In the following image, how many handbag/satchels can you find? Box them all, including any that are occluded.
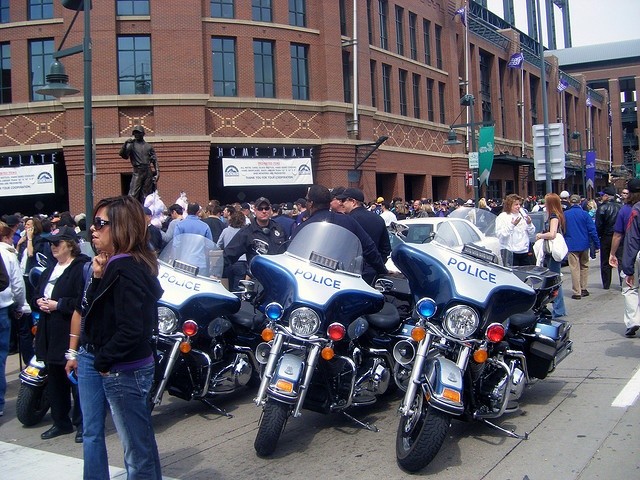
[543,226,563,255]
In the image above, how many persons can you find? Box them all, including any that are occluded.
[86,196,161,480]
[0,222,27,416]
[609,179,640,337]
[18,220,40,365]
[66,225,110,480]
[623,200,640,288]
[0,211,86,229]
[523,195,538,212]
[36,229,91,443]
[534,194,566,317]
[595,187,621,290]
[562,195,601,299]
[163,186,391,308]
[496,194,535,267]
[559,191,571,212]
[618,187,629,205]
[120,126,159,205]
[364,198,501,222]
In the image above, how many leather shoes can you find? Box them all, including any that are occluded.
[75,426,82,443]
[41,425,73,439]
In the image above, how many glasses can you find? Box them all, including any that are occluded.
[258,207,270,211]
[93,217,111,230]
[47,241,61,247]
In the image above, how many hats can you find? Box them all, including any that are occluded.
[377,197,384,203]
[133,125,144,134]
[336,188,365,202]
[7,215,20,226]
[42,226,77,242]
[598,187,615,196]
[570,194,582,203]
[255,197,270,207]
[53,211,60,217]
[560,190,570,198]
[272,204,280,213]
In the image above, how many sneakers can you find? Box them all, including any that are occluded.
[572,295,581,299]
[580,289,590,297]
[626,325,639,338]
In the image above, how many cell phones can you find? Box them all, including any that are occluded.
[67,370,78,385]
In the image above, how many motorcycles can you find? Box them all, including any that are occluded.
[12,251,53,428]
[153,233,268,420]
[391,234,572,472]
[250,220,412,455]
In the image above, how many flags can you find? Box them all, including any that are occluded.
[449,6,466,27]
[506,52,525,71]
[607,103,615,126]
[555,77,569,94]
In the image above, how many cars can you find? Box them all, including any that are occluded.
[385,218,504,274]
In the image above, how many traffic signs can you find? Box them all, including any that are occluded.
[532,123,567,181]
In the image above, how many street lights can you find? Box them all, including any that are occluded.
[35,0,94,240]
[565,132,586,198]
[442,94,478,208]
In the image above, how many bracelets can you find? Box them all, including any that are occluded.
[27,239,33,242]
[67,332,81,340]
[64,348,79,361]
